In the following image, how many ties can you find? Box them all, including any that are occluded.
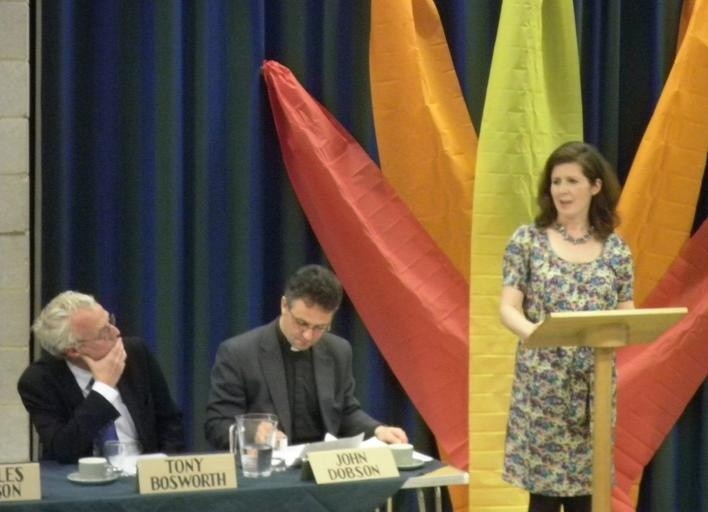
[87,378,119,456]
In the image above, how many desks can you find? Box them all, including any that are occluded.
[0,439,471,512]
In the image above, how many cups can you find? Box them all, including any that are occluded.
[76,455,108,478]
[103,438,143,472]
[391,444,414,465]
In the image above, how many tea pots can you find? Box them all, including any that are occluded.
[228,412,280,477]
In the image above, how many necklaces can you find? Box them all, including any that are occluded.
[555,223,595,245]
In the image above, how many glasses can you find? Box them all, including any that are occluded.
[77,313,116,342]
[291,312,329,332]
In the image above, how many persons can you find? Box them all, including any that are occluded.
[500,141,634,512]
[205,264,409,450]
[17,289,184,463]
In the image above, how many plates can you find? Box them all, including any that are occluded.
[395,458,424,472]
[67,468,119,486]
